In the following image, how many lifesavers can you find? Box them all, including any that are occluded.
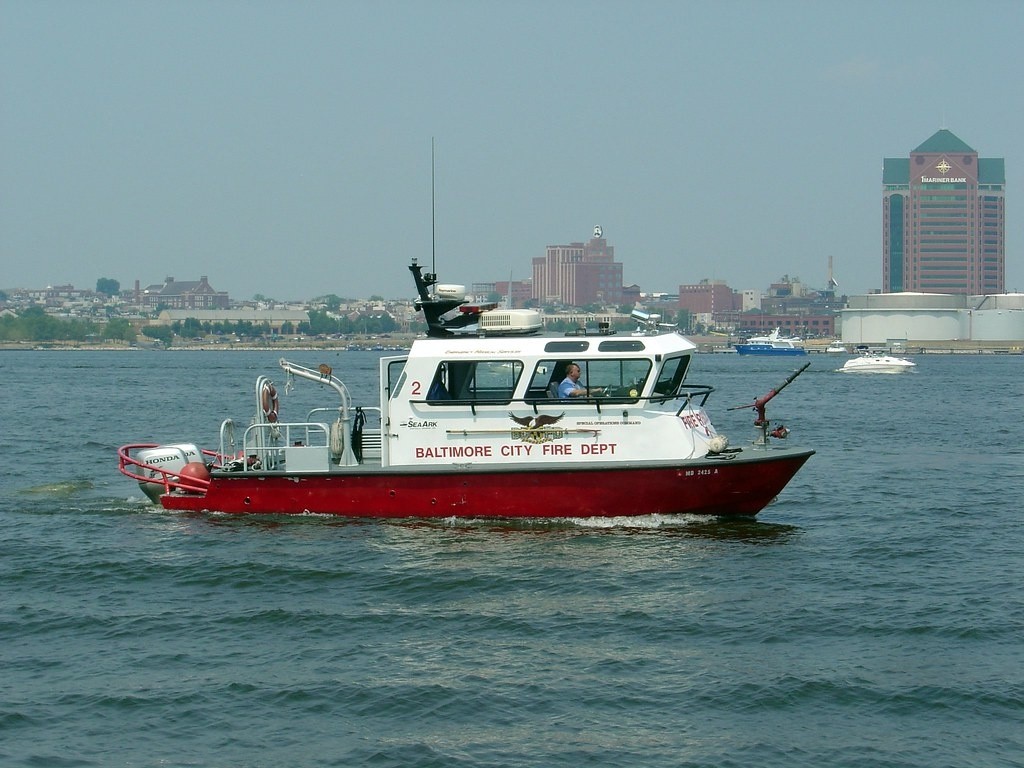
[262,384,279,421]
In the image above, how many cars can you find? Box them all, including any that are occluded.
[209,337,229,344]
[291,332,392,341]
[191,337,204,342]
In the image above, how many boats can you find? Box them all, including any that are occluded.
[842,342,917,375]
[117,136,816,520]
[827,339,847,353]
[731,324,808,357]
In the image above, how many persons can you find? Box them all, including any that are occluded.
[558,364,602,398]
